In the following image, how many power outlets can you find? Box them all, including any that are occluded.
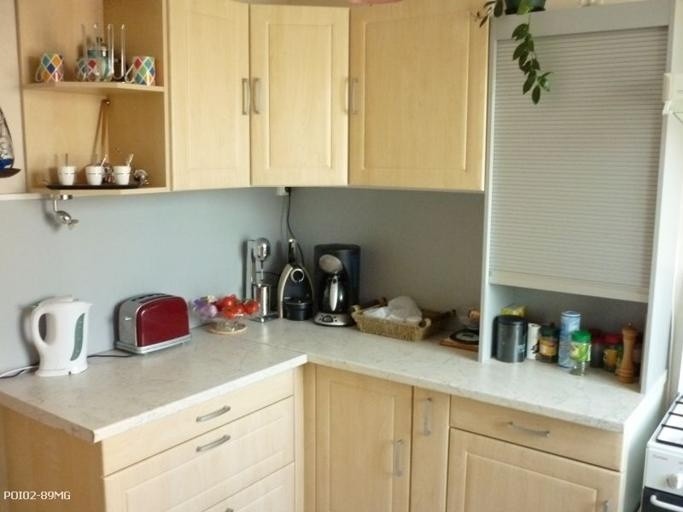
[277,186,293,197]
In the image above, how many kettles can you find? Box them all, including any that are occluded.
[24,294,92,376]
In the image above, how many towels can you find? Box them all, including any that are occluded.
[362,295,423,324]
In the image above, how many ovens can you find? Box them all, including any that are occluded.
[639,447,682,512]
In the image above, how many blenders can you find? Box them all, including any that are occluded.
[245,238,279,322]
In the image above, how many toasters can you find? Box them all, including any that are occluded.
[114,292,192,354]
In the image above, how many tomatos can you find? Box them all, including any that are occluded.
[216,296,259,315]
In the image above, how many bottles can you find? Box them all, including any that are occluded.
[558,311,581,367]
[616,322,637,378]
[632,332,644,376]
[588,329,603,367]
[539,327,558,362]
[570,332,589,376]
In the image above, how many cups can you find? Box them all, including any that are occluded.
[74,56,104,80]
[84,166,103,186]
[36,52,65,83]
[124,55,157,86]
[58,166,73,184]
[113,164,131,184]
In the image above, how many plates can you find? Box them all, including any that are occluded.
[450,329,479,344]
[81,185,111,188]
[46,184,81,189]
[112,184,138,190]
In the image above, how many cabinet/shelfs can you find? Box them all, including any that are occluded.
[17,0,171,197]
[104,0,347,193]
[348,0,489,194]
[0,355,308,512]
[306,353,667,512]
[477,1,683,393]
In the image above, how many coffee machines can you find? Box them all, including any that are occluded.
[313,245,360,327]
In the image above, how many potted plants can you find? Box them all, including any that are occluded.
[475,1,552,106]
